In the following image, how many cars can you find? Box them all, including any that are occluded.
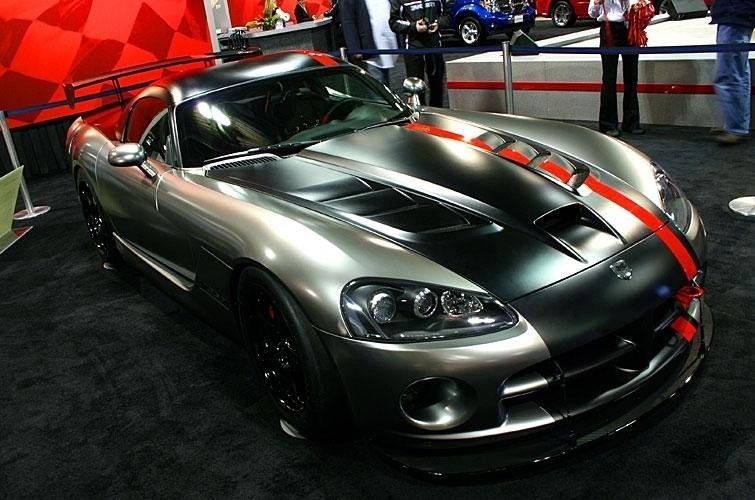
[438,2,535,45]
[532,1,602,28]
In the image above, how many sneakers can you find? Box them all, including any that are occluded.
[709,129,746,143]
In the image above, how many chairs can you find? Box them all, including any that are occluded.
[265,79,326,156]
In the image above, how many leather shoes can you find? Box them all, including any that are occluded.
[631,129,643,133]
[607,130,619,135]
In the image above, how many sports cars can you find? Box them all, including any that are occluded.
[63,41,716,481]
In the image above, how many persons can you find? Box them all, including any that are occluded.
[706,0,755,144]
[338,0,400,87]
[587,0,645,136]
[389,0,454,109]
[318,0,347,50]
[262,0,317,27]
